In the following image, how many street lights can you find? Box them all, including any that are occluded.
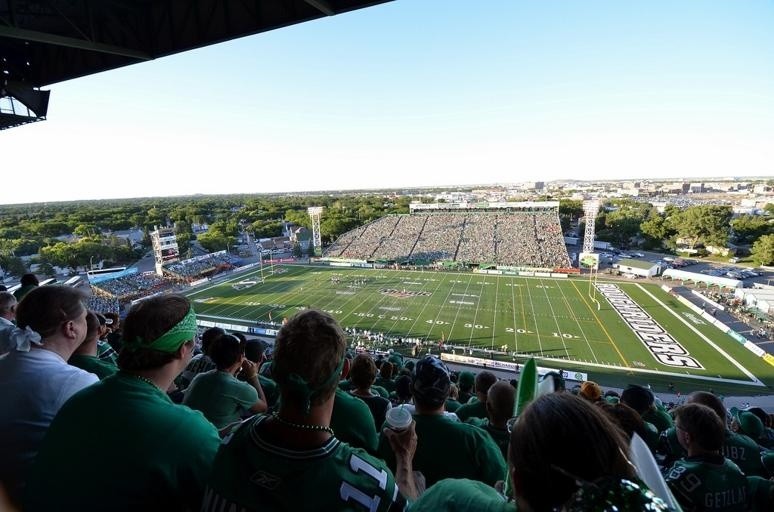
[89,256,95,280]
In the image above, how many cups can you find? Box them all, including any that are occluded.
[382,407,412,431]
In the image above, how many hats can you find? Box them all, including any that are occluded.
[729,406,765,440]
[457,370,475,387]
[412,354,451,404]
[580,380,602,400]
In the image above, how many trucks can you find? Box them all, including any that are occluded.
[564,236,581,247]
[593,240,615,252]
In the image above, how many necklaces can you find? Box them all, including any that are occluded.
[272,410,335,436]
[131,374,160,389]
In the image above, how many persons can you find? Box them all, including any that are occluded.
[3,287,102,508]
[67,311,124,381]
[698,283,772,338]
[209,311,417,512]
[18,295,221,509]
[295,309,773,512]
[267,311,273,321]
[3,275,39,355]
[328,276,340,284]
[79,277,206,316]
[164,327,280,440]
[349,278,369,292]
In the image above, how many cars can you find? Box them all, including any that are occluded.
[622,272,638,279]
[597,246,647,261]
[63,272,68,277]
[145,253,152,258]
[698,263,765,281]
[728,257,742,263]
[653,256,702,270]
[259,246,294,257]
